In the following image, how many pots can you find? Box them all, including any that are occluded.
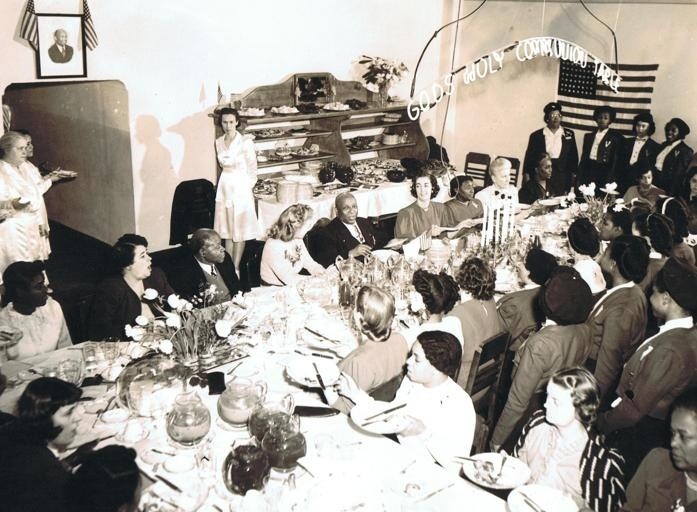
[382,132,399,146]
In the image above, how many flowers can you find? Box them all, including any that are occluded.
[559,178,620,226]
[119,283,255,395]
[358,54,411,105]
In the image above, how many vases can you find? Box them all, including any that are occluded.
[377,79,388,108]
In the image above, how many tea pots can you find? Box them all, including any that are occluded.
[162,368,306,498]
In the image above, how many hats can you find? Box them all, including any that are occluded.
[672,117,690,138]
[633,113,653,123]
[538,265,595,326]
[662,255,696,311]
[544,102,562,112]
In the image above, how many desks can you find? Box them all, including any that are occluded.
[254,156,467,244]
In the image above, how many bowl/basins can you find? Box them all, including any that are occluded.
[423,168,443,175]
[386,170,406,183]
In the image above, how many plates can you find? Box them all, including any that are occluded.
[254,193,273,200]
[276,179,313,205]
[0,325,22,346]
[284,352,577,511]
[238,113,266,119]
[271,112,301,117]
[292,151,317,158]
[321,108,348,113]
[255,134,283,138]
[287,131,306,136]
[84,398,164,464]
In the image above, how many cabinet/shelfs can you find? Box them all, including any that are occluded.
[208,72,430,186]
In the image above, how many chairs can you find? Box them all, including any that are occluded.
[0,152,522,344]
[363,274,671,500]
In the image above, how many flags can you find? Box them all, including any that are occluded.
[557,61,660,137]
[20,0,39,52]
[83,0,99,50]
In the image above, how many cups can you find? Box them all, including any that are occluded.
[334,232,524,328]
[56,334,120,386]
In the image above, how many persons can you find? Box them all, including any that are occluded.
[49,29,73,63]
[3,103,697,512]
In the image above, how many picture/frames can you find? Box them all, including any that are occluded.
[34,12,88,76]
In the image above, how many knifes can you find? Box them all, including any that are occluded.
[303,326,336,343]
